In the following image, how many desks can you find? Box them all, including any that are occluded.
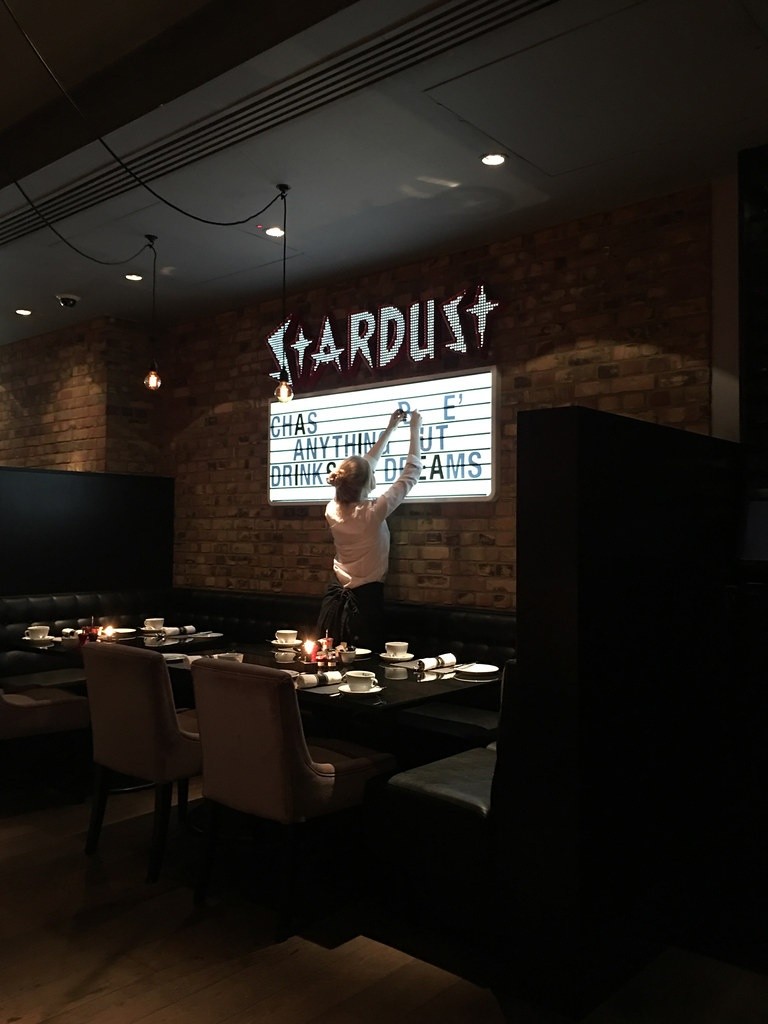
[109,633,503,845]
[6,610,215,792]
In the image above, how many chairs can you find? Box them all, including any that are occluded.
[80,637,200,880]
[187,656,401,944]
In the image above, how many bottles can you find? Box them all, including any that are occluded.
[328,652,336,670]
[316,652,325,673]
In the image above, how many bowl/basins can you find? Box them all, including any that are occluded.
[355,648,372,659]
[113,629,136,637]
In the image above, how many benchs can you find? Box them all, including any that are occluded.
[0,586,511,948]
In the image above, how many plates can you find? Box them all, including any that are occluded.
[337,685,381,694]
[380,653,414,662]
[22,636,54,640]
[112,637,136,640]
[276,661,295,663]
[141,628,163,632]
[271,640,302,648]
[454,663,499,674]
[188,633,223,637]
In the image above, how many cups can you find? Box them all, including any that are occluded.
[144,618,164,629]
[144,637,158,645]
[275,630,298,644]
[346,671,378,691]
[218,653,243,664]
[275,652,295,661]
[25,626,50,639]
[385,642,408,657]
[340,652,356,663]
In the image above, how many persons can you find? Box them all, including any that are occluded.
[309,409,422,755]
